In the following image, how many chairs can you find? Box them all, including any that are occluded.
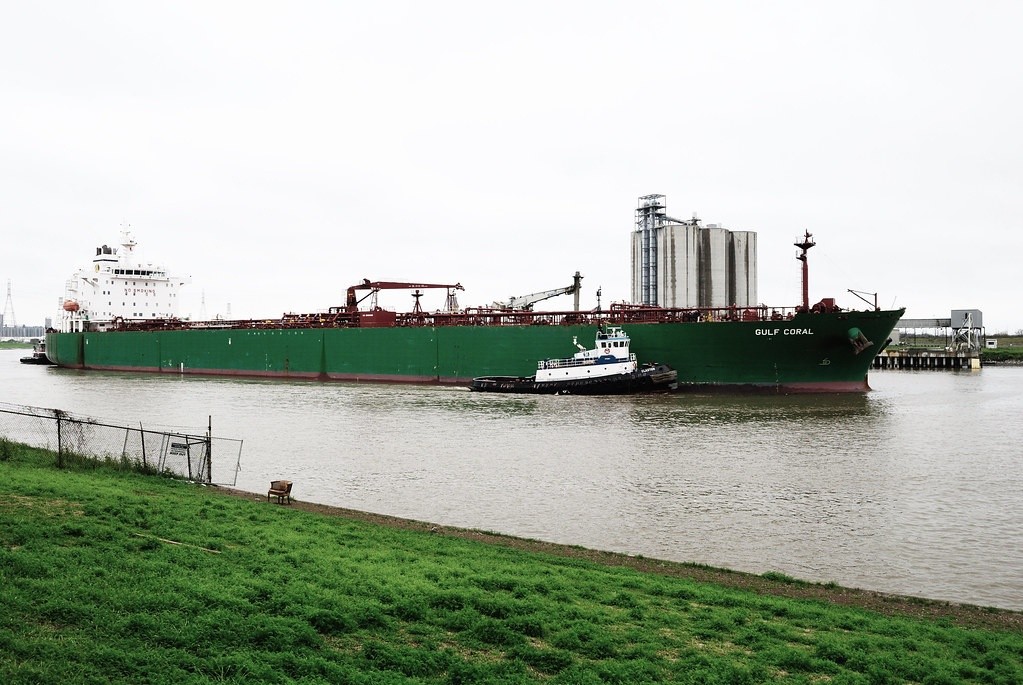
[268,479,292,505]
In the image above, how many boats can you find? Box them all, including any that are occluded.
[467,323,680,395]
[42,221,906,392]
[18,338,54,365]
